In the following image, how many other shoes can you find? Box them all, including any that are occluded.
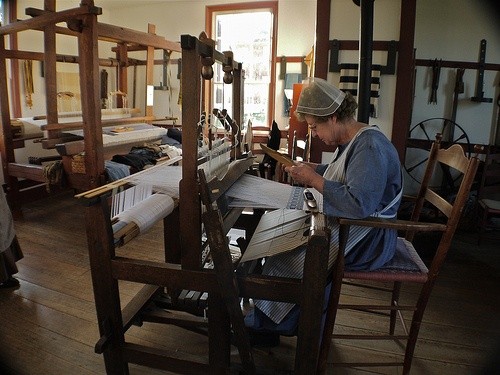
[198,300,208,317]
[229,326,279,347]
[4,278,19,287]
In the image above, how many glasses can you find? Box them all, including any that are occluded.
[309,115,325,131]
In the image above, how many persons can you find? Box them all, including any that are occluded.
[0,183,25,287]
[230,76,405,348]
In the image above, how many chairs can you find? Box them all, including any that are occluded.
[248,120,281,180]
[290,130,309,187]
[474,164,500,247]
[317,133,484,375]
